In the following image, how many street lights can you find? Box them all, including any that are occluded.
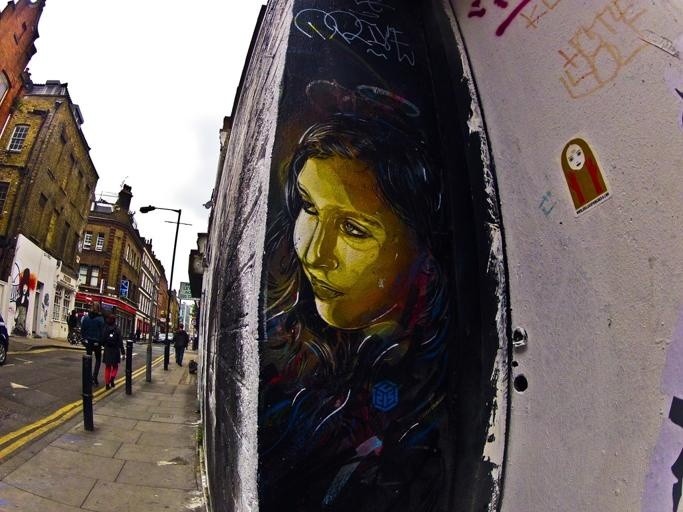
[139,206,181,345]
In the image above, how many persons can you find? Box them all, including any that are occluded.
[80,302,103,387]
[265,110,461,511]
[67,308,78,345]
[14,277,30,314]
[102,314,126,390]
[172,323,188,364]
[74,310,89,327]
[135,327,140,342]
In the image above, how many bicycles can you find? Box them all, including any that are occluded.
[67,327,80,345]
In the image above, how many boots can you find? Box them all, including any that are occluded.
[106,377,115,389]
[92,375,100,384]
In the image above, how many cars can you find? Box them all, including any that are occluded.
[155,333,166,343]
[167,332,174,343]
[0,314,8,363]
